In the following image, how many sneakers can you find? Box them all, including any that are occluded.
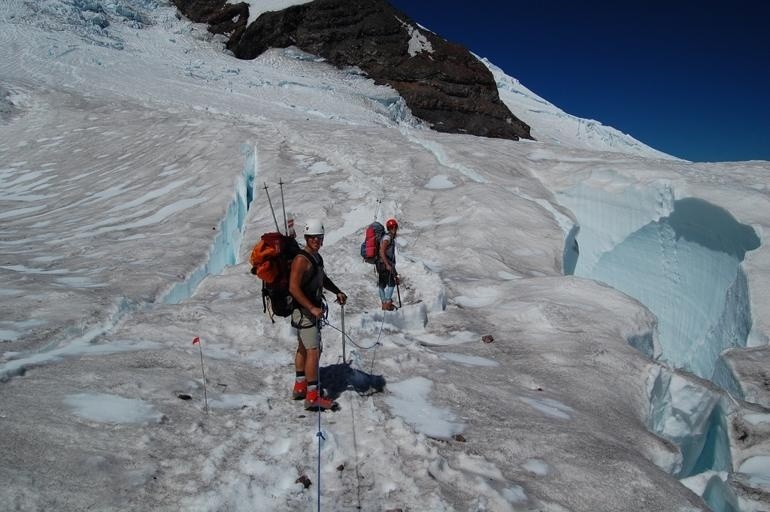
[382,303,398,310]
[294,380,339,408]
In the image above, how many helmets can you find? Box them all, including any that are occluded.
[304,220,324,235]
[386,219,398,230]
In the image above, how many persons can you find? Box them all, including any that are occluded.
[285,219,346,409]
[376,219,400,312]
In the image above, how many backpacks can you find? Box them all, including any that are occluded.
[250,233,324,317]
[360,222,393,264]
